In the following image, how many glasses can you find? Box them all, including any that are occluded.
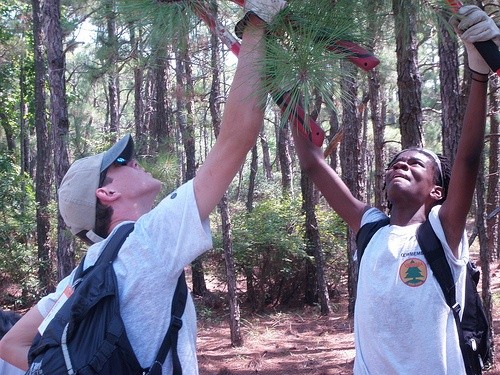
[57,133,135,236]
[99,154,128,188]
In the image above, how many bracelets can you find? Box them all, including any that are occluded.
[471,77,489,83]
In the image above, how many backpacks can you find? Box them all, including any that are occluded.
[24,222,188,375]
[354,217,494,374]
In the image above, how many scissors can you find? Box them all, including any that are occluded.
[187,0,380,147]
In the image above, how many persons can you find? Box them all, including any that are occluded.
[288,6,500,375]
[0,0,289,374]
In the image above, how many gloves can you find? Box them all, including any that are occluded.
[448,4,500,75]
[234,0,288,40]
